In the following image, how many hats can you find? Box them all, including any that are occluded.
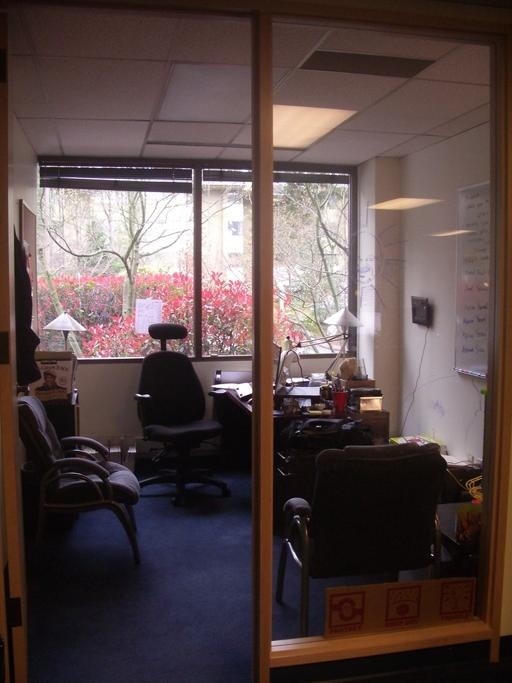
[43,370,56,376]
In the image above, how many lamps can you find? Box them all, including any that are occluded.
[276,304,364,408]
[43,310,88,350]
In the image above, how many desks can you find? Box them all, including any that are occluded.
[219,372,390,530]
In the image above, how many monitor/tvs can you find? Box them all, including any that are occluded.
[235,340,282,400]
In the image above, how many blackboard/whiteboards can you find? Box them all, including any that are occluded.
[452,180,491,379]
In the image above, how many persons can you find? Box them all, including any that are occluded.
[34,370,68,391]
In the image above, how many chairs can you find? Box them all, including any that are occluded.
[136,322,237,507]
[276,441,449,637]
[17,395,146,563]
